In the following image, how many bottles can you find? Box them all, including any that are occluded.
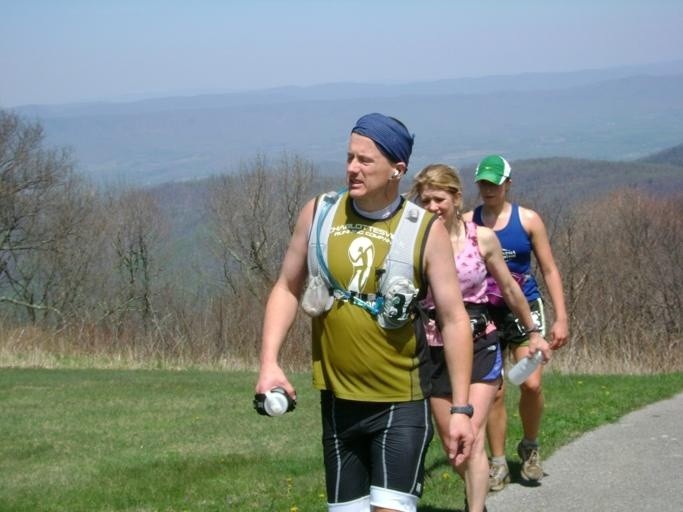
[507,350,547,387]
[261,388,295,417]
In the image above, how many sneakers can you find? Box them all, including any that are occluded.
[517,440,543,482]
[489,460,511,491]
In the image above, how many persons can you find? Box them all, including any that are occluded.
[254,113,476,512]
[460,154,571,493]
[408,162,553,512]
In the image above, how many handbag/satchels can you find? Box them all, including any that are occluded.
[430,301,491,338]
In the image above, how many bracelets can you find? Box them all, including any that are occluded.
[523,324,539,334]
[448,403,474,419]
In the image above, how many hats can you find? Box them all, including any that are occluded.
[474,155,511,185]
